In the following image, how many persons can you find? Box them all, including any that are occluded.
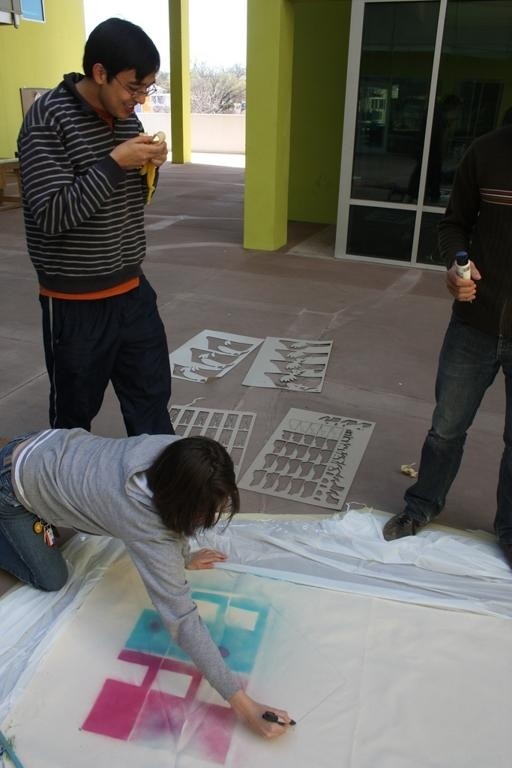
[382,120,512,572]
[0,426,292,741]
[401,117,451,263]
[16,18,177,437]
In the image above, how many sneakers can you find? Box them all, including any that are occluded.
[383,511,422,540]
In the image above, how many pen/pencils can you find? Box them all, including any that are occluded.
[454,252,471,301]
[263,711,297,724]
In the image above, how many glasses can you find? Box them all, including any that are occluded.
[114,77,157,97]
